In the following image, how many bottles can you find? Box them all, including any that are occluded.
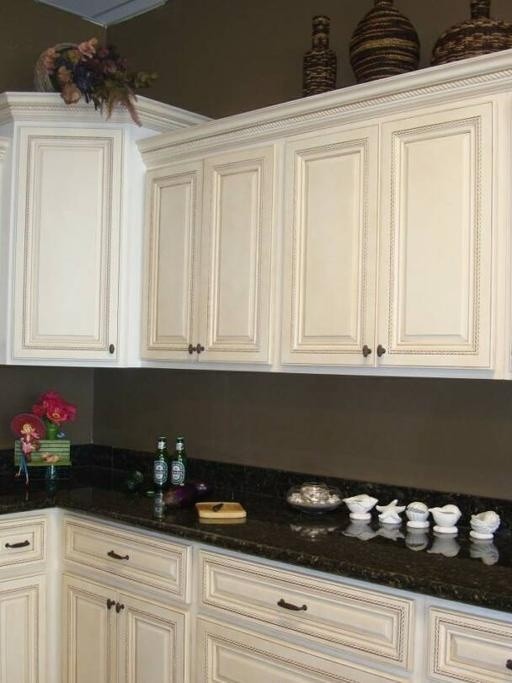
[169,431,188,490]
[153,491,168,521]
[153,432,168,490]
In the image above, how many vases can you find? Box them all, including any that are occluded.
[46,422,58,436]
[302,15,337,97]
[32,42,79,92]
[430,0,512,67]
[349,0,421,84]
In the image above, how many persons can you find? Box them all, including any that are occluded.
[11,412,45,485]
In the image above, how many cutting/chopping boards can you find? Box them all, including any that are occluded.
[194,502,247,518]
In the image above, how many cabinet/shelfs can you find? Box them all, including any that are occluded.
[284,51,503,381]
[60,519,193,683]
[195,545,420,683]
[0,91,128,370]
[0,515,53,683]
[140,103,284,373]
[422,602,512,683]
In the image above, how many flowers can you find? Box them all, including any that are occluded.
[31,388,79,440]
[43,37,161,128]
